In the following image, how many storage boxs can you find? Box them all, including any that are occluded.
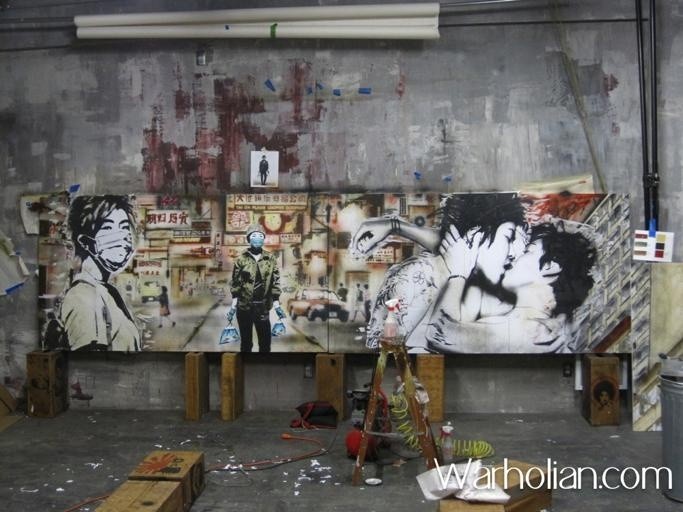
[94,450,205,512]
[439,460,554,512]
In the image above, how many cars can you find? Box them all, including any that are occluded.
[287,289,348,322]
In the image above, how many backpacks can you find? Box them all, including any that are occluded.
[41,268,112,351]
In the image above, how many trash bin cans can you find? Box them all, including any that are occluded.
[659,375,683,502]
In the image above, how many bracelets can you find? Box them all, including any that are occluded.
[387,214,400,233]
[445,275,468,280]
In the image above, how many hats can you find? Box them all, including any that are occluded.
[247,224,267,238]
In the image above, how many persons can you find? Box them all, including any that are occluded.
[346,213,597,356]
[257,153,269,185]
[361,283,371,323]
[361,193,574,357]
[348,281,365,322]
[593,381,614,407]
[156,285,177,329]
[223,222,286,352]
[44,194,147,355]
[337,282,348,302]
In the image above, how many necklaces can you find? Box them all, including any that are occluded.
[512,304,552,318]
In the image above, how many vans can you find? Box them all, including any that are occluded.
[140,275,163,302]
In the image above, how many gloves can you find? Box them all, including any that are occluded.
[227,308,236,320]
[275,307,286,318]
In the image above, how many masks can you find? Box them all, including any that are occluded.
[77,231,135,273]
[250,238,264,248]
